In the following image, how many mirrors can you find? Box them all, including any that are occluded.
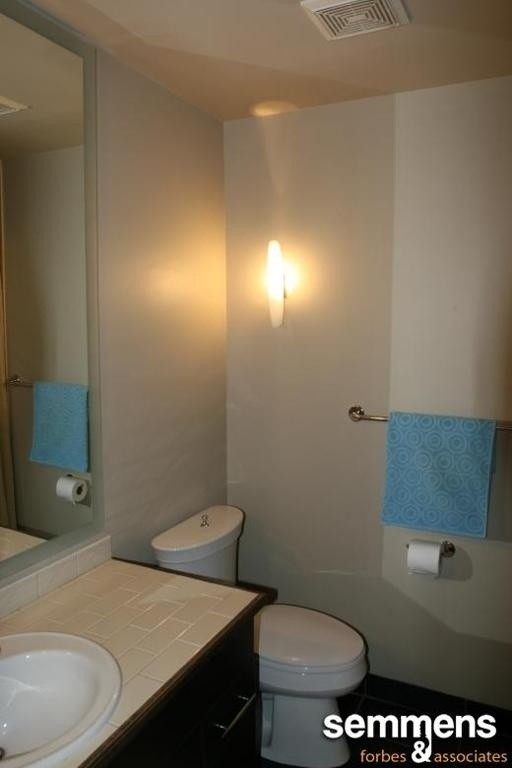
[1,0,107,592]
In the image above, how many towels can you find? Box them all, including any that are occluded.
[378,410,498,539]
[29,379,91,474]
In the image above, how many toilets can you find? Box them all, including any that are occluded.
[151,505,369,768]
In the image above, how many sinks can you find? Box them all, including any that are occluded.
[1,631,122,766]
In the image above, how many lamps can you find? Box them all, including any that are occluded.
[265,239,288,327]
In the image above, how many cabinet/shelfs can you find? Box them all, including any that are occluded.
[106,618,265,767]
